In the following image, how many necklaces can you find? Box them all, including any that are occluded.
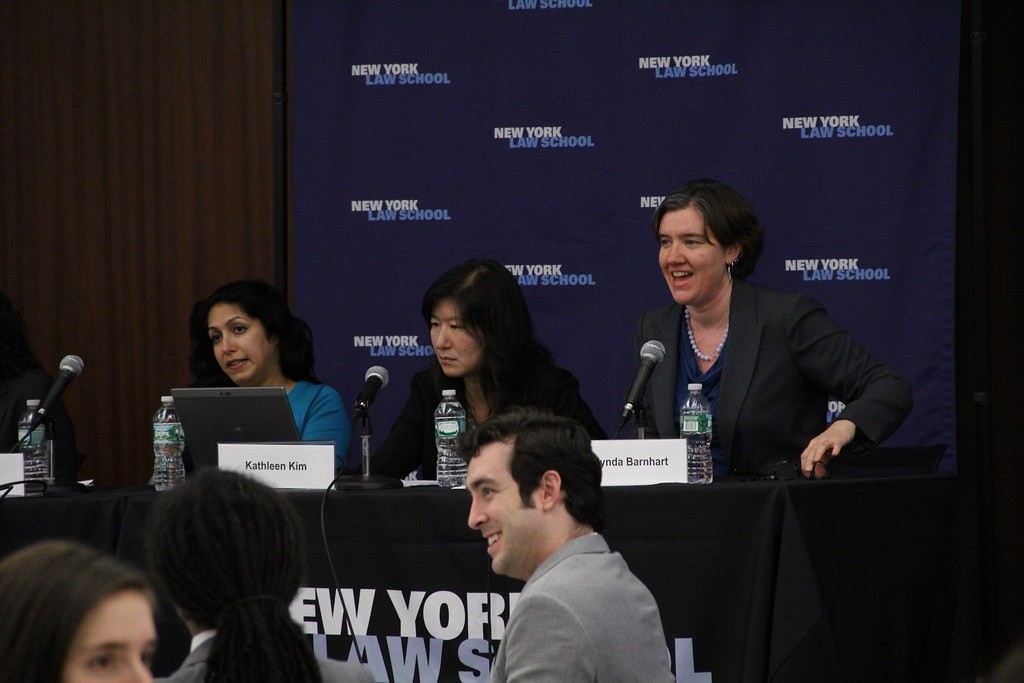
[684,308,730,361]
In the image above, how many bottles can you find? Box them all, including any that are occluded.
[682,382,716,485]
[431,389,469,485]
[17,397,49,484]
[151,394,184,493]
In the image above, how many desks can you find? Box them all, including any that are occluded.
[0,474,972,682]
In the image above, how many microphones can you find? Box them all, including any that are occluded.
[352,365,389,425]
[30,355,86,431]
[616,340,666,428]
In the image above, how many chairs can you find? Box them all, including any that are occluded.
[862,443,947,475]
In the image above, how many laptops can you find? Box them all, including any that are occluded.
[170,386,299,473]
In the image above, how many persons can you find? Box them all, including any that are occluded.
[458,407,677,681]
[145,468,380,682]
[629,180,914,480]
[0,540,158,683]
[351,258,611,484]
[146,275,351,491]
[1,290,79,488]
